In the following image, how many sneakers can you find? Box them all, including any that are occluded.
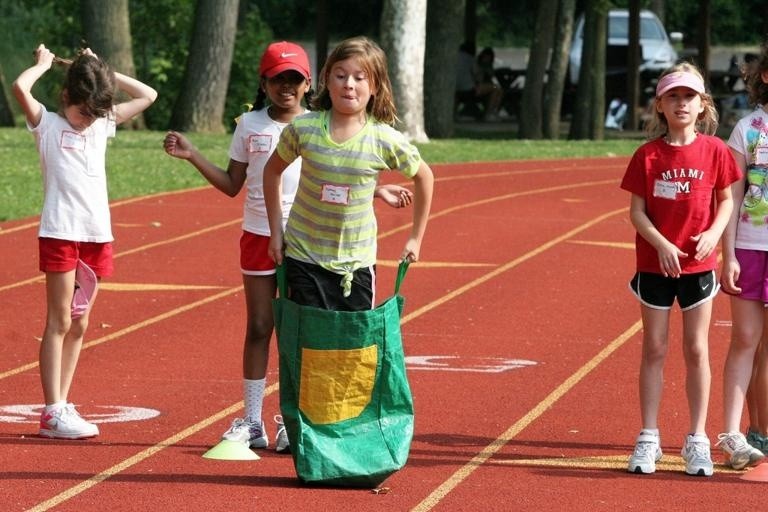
[274,414,291,454]
[628,427,768,477]
[222,416,268,448]
[39,403,99,439]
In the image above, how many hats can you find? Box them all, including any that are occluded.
[260,41,309,83]
[71,259,97,320]
[655,72,705,96]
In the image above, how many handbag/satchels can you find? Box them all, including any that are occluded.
[273,298,414,487]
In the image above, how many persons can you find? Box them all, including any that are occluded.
[474,46,503,123]
[719,49,768,470]
[11,43,159,439]
[722,53,757,110]
[262,36,435,487]
[454,39,479,123]
[163,39,415,453]
[619,62,745,476]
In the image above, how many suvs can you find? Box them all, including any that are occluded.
[569,7,678,90]
